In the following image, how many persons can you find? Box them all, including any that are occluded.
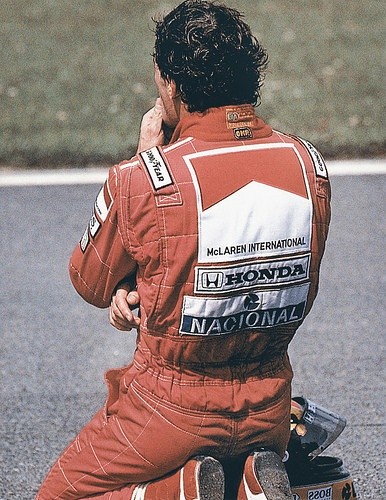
[37,0,333,500]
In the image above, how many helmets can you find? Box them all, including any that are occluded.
[289,396,356,500]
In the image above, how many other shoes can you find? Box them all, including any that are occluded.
[181,454,225,500]
[242,449,294,499]
